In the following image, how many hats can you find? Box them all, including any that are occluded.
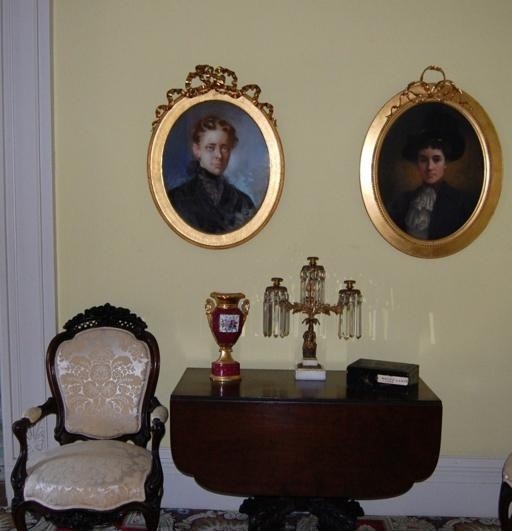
[401,127,463,164]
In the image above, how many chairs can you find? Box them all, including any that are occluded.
[9,304,168,531]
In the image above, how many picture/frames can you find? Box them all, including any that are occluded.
[147,65,285,251]
[358,66,502,262]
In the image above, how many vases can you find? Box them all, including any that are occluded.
[206,292,250,383]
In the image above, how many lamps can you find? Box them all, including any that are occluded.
[264,256,363,383]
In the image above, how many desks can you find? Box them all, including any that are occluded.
[168,368,442,531]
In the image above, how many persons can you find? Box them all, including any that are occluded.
[164,113,255,234]
[389,128,475,241]
